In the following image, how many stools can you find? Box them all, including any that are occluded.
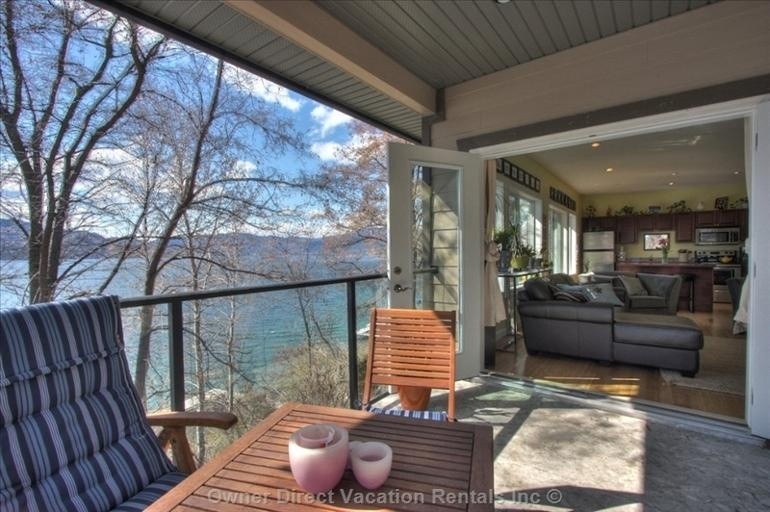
[670,272,697,314]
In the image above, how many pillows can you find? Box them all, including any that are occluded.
[581,282,625,307]
[618,274,649,296]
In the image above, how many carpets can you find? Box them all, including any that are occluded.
[659,335,745,396]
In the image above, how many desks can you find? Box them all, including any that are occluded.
[493,265,554,354]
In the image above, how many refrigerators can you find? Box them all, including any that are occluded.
[581,230,615,272]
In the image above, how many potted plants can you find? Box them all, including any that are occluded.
[492,223,553,273]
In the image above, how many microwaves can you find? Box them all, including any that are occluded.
[696,227,741,246]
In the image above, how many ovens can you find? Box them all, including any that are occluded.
[712,263,743,304]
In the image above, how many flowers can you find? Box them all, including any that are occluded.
[658,239,671,247]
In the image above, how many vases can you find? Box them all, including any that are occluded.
[662,247,669,263]
[397,386,431,411]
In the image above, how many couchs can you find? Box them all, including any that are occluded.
[517,269,704,379]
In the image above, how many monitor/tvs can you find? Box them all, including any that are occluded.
[643,233,670,251]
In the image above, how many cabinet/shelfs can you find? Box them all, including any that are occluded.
[739,209,748,240]
[675,213,695,242]
[637,214,674,231]
[587,217,616,230]
[695,209,738,227]
[617,215,637,242]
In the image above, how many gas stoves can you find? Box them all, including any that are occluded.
[698,255,739,265]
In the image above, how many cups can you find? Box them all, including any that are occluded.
[286,421,350,497]
[350,440,394,491]
